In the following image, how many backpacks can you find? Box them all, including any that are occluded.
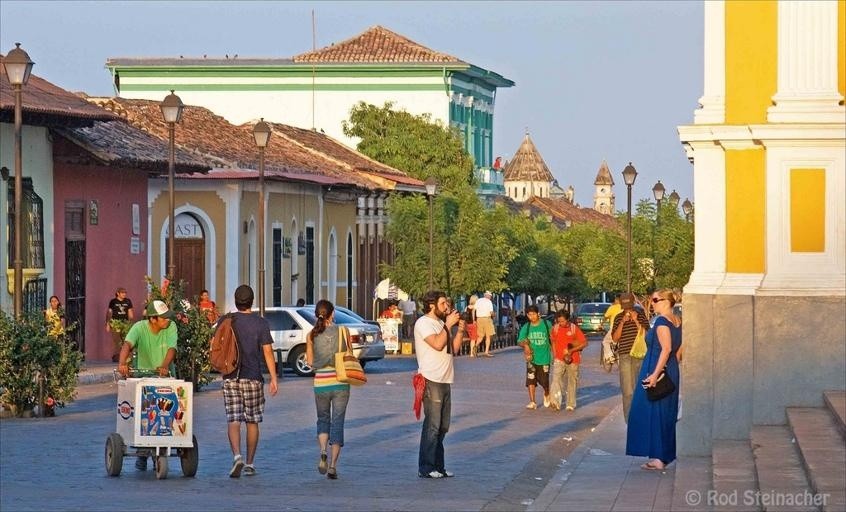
[209,313,240,375]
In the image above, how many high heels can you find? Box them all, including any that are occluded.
[317,450,327,475]
[326,467,339,478]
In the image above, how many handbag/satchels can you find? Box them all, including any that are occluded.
[334,326,367,386]
[630,324,648,359]
[646,318,675,401]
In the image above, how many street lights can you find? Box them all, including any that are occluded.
[682,198,694,227]
[653,180,664,291]
[159,88,185,310]
[424,175,438,293]
[4,40,34,322]
[668,189,680,222]
[253,116,272,319]
[622,162,638,295]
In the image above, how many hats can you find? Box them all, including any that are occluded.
[619,292,636,309]
[484,289,493,297]
[146,300,178,322]
[235,284,253,303]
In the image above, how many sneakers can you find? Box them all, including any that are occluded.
[134,456,146,472]
[418,469,444,479]
[566,405,576,414]
[527,402,538,411]
[229,456,244,478]
[153,460,159,470]
[439,467,453,478]
[542,396,550,407]
[550,402,561,415]
[243,466,258,476]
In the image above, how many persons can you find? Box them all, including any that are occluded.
[465,295,478,358]
[550,310,588,410]
[415,291,464,478]
[517,305,552,409]
[626,287,683,470]
[398,299,416,339]
[296,299,303,307]
[200,290,220,328]
[383,304,400,319]
[106,288,134,362]
[211,286,277,478]
[612,294,650,424]
[45,296,66,336]
[119,301,178,469]
[307,300,353,478]
[473,291,496,357]
[599,294,624,366]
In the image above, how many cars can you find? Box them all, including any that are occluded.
[574,303,611,336]
[212,307,385,377]
[305,305,381,328]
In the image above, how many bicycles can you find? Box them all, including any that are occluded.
[600,327,619,373]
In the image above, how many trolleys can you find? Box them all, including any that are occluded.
[105,368,198,480]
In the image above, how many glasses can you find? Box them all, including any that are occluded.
[652,296,669,303]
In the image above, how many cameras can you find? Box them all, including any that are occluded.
[450,307,476,326]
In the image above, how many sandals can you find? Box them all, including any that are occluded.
[473,346,479,355]
[485,353,493,356]
[641,459,669,472]
